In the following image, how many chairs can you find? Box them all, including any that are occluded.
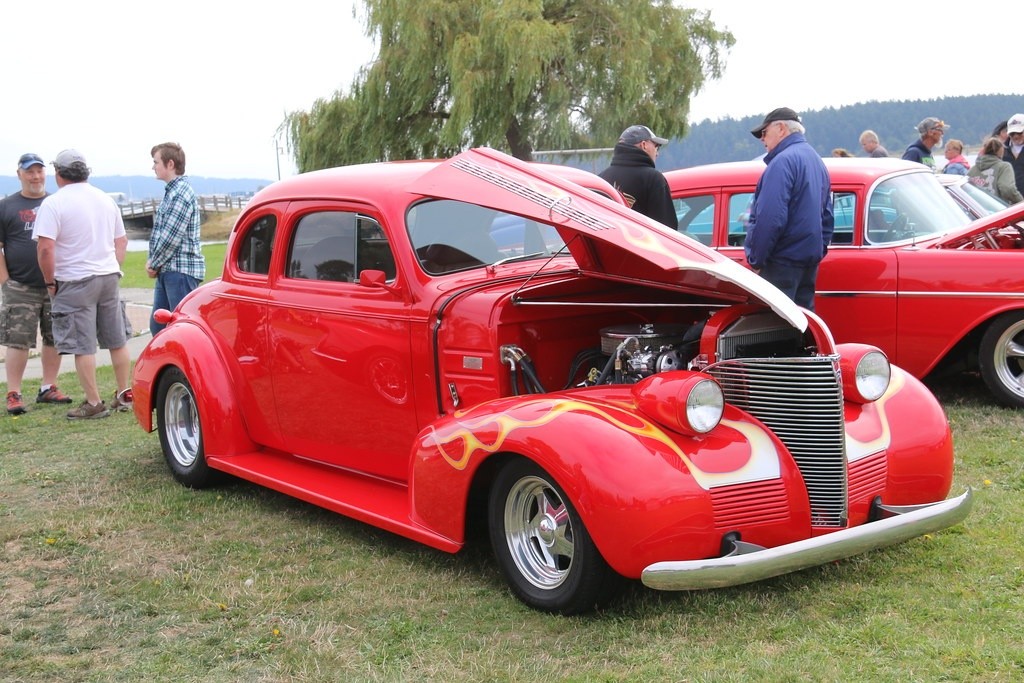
[292,212,509,283]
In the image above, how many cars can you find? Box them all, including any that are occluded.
[660,158,1023,409]
[132,146,973,616]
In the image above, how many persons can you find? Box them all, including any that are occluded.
[594,124,677,234]
[967,138,1023,205]
[743,107,835,310]
[0,152,73,413]
[832,148,854,157]
[977,121,1008,159]
[31,150,132,419]
[895,117,950,233]
[1001,114,1024,198]
[941,139,971,175]
[145,142,207,339]
[859,130,888,157]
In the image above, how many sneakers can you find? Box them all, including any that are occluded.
[7,390,23,412]
[67,400,109,420]
[36,388,72,403]
[110,391,132,408]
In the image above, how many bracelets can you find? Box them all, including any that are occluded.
[44,282,55,286]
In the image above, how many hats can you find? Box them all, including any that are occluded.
[50,148,86,168]
[918,117,951,132]
[618,125,669,145]
[17,153,45,169]
[751,107,800,138]
[1007,114,1024,135]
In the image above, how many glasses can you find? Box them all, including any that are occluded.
[932,121,944,129]
[1010,133,1023,136]
[935,128,943,135]
